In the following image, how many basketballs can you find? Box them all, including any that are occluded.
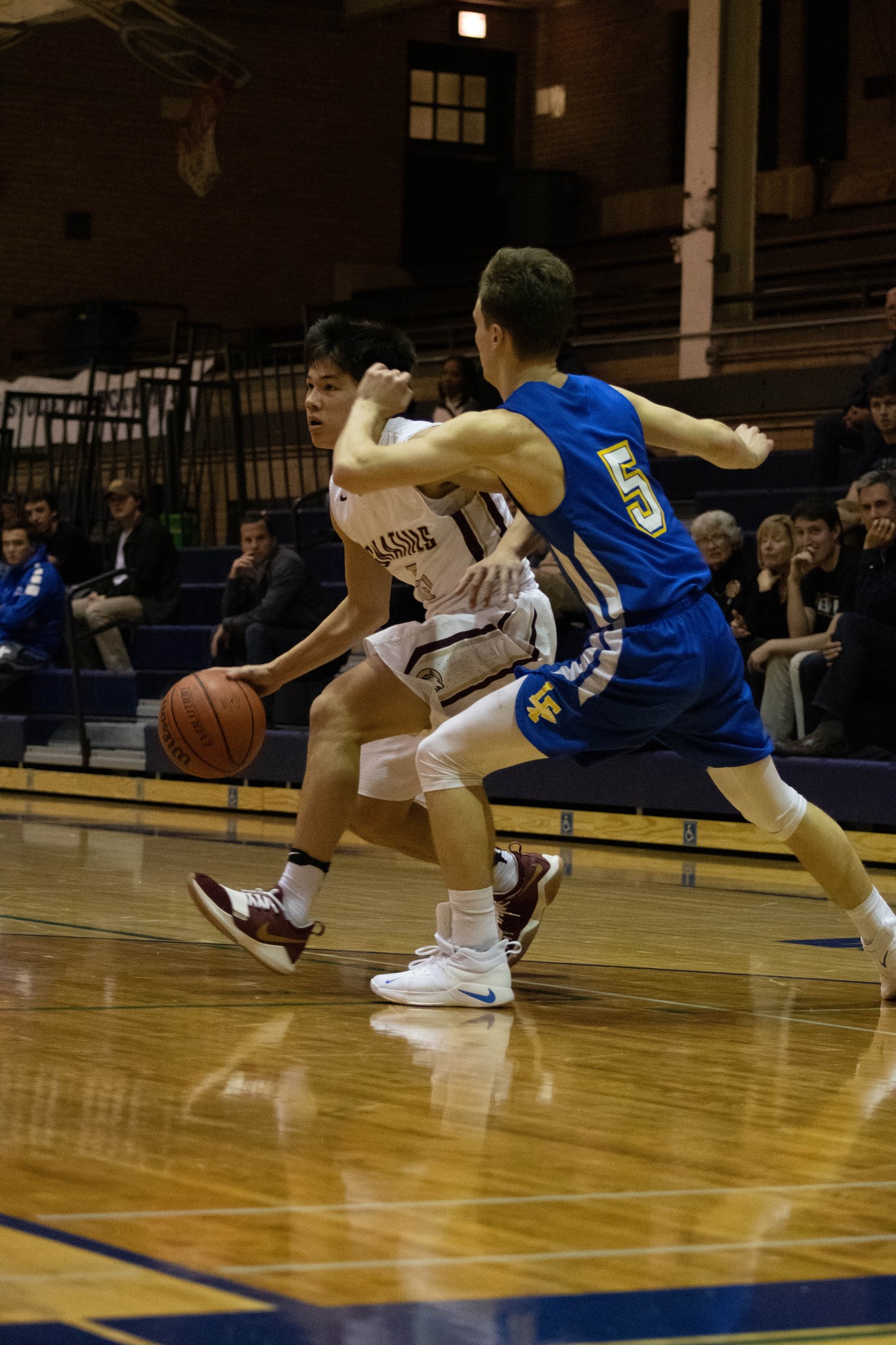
[157,671,267,778]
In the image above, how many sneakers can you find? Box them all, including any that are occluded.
[371,932,523,1009]
[861,916,896,1002]
[369,1006,514,1071]
[493,842,566,969]
[187,872,324,974]
[187,1014,305,1121]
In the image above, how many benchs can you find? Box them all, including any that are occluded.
[0,442,896,834]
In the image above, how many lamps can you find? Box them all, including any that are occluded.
[450,10,487,39]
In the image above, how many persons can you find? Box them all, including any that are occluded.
[505,494,584,620]
[187,324,562,973]
[0,479,180,672]
[690,290,895,759]
[433,354,477,416]
[333,248,895,1006]
[210,514,325,729]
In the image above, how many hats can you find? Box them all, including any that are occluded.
[104,478,141,498]
